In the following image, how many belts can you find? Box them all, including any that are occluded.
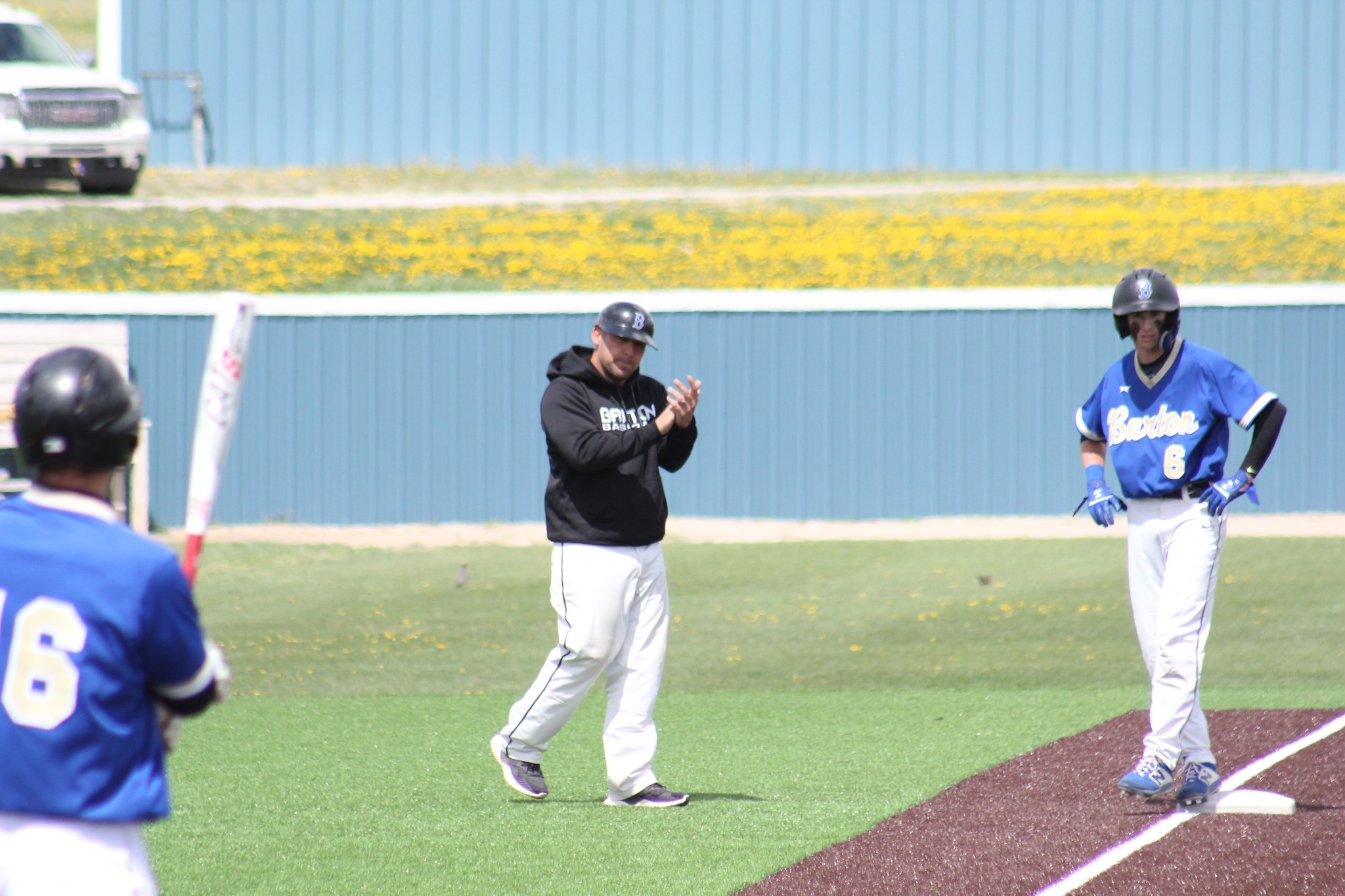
[1162,482,1210,499]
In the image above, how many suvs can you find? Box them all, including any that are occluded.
[0,3,150,196]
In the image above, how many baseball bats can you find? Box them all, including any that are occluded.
[155,297,254,738]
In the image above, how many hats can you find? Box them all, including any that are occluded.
[596,302,657,349]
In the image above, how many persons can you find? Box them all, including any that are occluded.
[1075,268,1288,804]
[0,348,227,896]
[490,302,702,807]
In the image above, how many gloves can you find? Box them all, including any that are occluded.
[1198,470,1260,518]
[1073,478,1127,530]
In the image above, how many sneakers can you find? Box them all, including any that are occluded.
[1176,758,1222,806]
[490,735,549,799]
[603,782,690,808]
[1117,747,1175,801]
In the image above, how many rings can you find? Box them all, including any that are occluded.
[681,401,686,405]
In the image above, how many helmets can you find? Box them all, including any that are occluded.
[13,347,140,471]
[1111,267,1180,340]
